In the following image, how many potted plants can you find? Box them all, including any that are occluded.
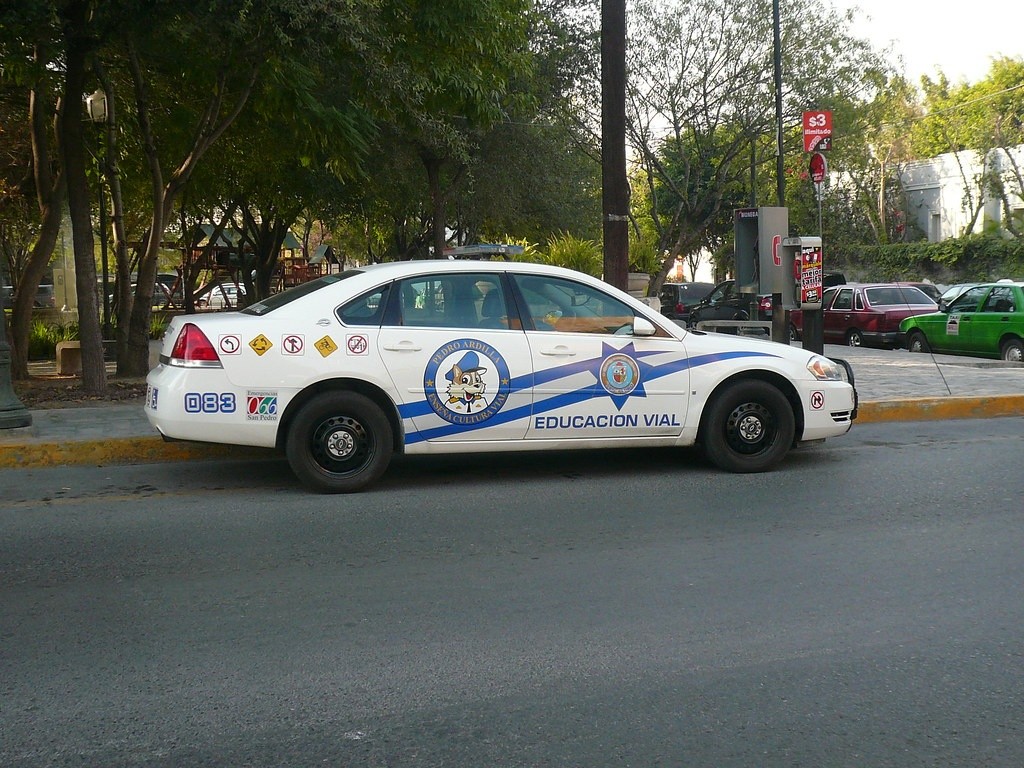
[599,239,665,291]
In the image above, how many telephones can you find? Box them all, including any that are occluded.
[794,258,801,280]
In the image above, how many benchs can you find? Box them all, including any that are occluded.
[695,320,772,341]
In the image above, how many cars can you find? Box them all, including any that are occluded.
[912,282,982,304]
[366,290,403,306]
[898,278,1024,362]
[108,281,172,308]
[197,283,246,307]
[143,243,858,493]
[790,282,942,350]
[690,278,772,332]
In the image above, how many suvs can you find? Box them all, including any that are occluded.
[659,281,727,325]
[5,285,56,308]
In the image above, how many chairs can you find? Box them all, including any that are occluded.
[477,288,507,329]
[994,299,1010,312]
